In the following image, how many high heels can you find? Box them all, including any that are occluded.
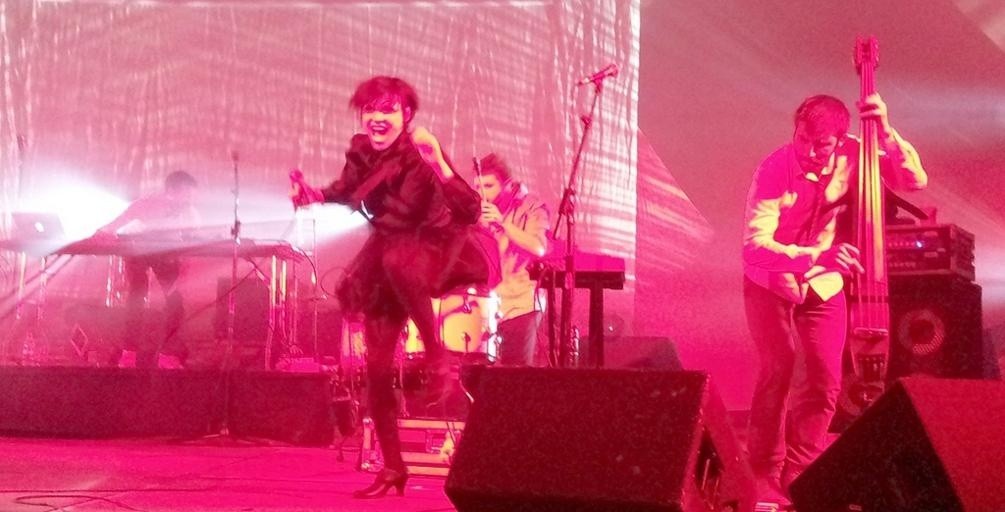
[352,467,408,498]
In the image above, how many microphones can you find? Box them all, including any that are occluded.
[577,64,617,84]
[231,150,239,172]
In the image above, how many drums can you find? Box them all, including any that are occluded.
[341,310,419,368]
[402,286,504,366]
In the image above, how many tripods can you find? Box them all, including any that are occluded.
[183,176,270,446]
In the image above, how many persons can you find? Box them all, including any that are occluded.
[95,171,198,368]
[474,153,550,366]
[742,94,929,507]
[290,76,490,499]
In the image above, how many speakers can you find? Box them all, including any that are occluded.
[785,378,1005,511]
[826,282,984,431]
[444,368,759,512]
